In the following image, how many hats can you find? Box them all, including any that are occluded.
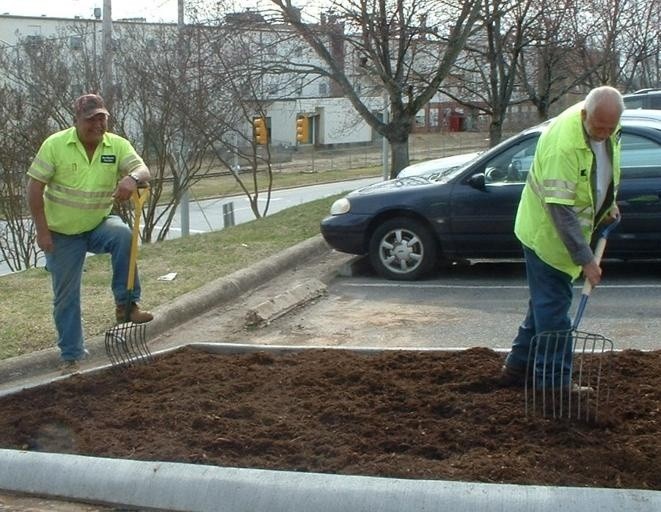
[72,93,112,121]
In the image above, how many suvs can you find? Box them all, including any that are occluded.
[621,88,661,111]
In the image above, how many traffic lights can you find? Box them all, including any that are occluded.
[253,116,268,145]
[296,116,309,145]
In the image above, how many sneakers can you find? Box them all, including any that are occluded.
[60,359,80,377]
[499,359,596,397]
[114,300,153,324]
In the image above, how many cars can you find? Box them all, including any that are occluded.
[394,109,661,183]
[319,120,661,282]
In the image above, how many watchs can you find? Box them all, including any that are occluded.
[125,170,142,184]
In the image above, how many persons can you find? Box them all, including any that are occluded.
[24,91,157,376]
[495,84,628,399]
